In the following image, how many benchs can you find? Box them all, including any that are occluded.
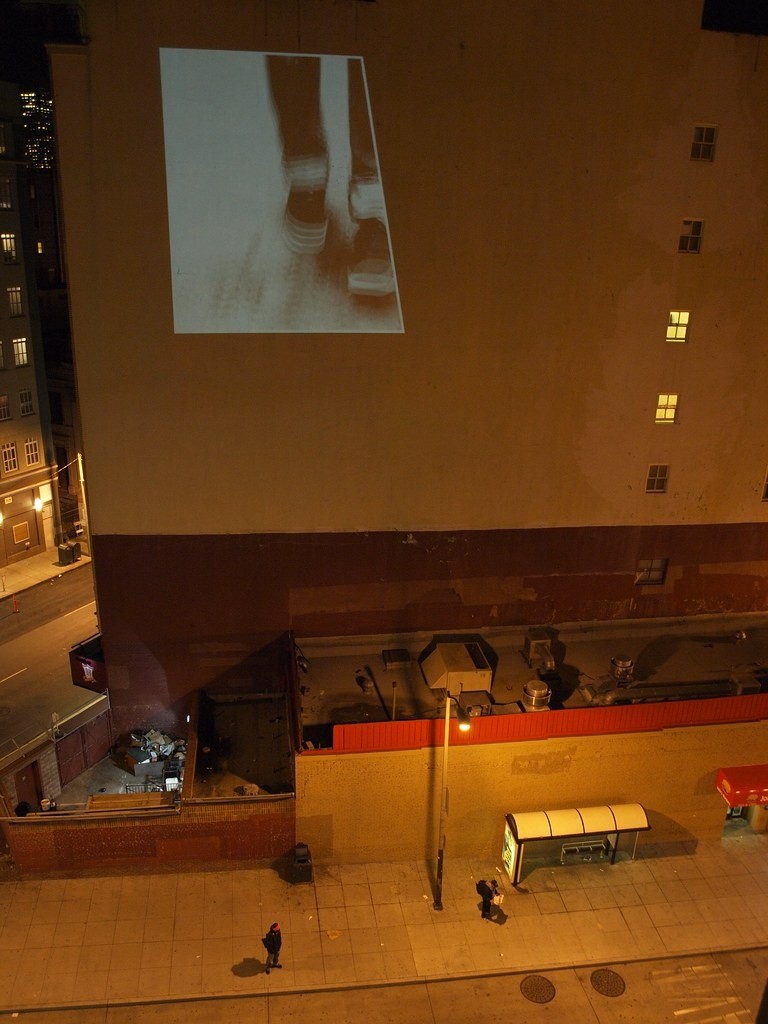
[560,838,608,863]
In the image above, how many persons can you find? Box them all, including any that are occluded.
[476,879,501,921]
[261,923,282,973]
[264,54,393,298]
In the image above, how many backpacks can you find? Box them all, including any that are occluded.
[262,933,269,948]
[476,880,488,895]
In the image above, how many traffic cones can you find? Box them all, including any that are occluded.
[11,594,20,614]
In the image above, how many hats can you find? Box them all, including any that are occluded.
[491,880,499,887]
[273,923,280,930]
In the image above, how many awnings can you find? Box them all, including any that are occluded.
[716,764,768,807]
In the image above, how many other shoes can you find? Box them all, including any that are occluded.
[282,187,330,257]
[266,966,270,975]
[482,917,489,921]
[347,218,395,296]
[272,964,282,968]
[485,911,494,915]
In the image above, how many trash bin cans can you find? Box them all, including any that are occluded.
[68,542,82,562]
[58,544,72,566]
[290,844,315,884]
